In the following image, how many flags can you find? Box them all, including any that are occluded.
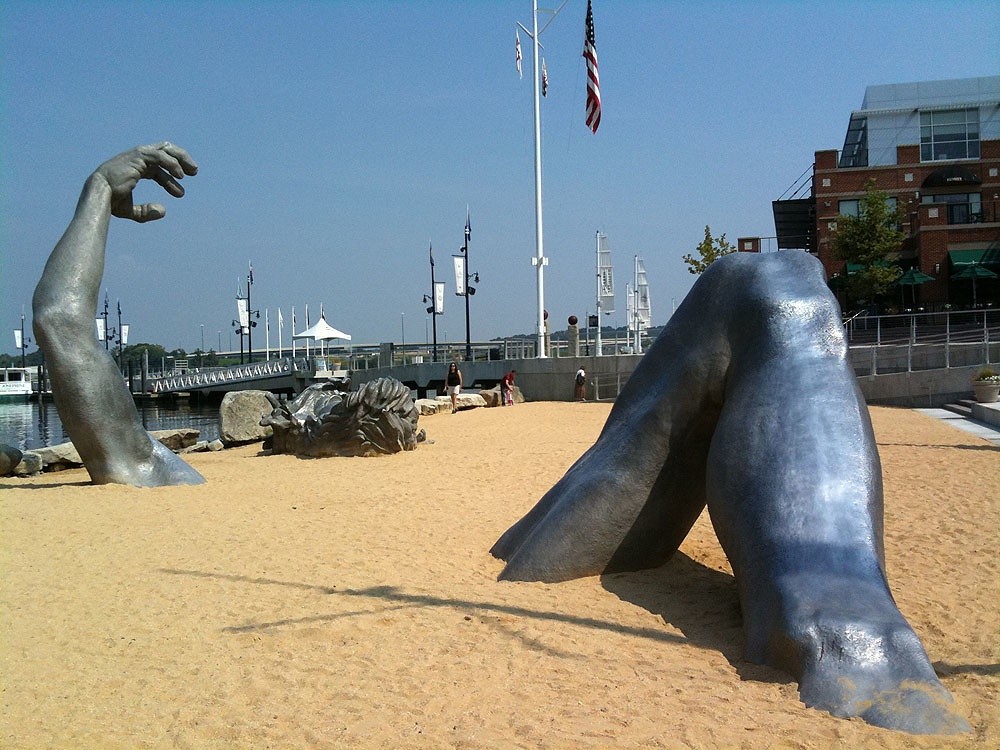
[579,0,602,135]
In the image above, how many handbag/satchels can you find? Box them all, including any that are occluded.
[578,373,585,385]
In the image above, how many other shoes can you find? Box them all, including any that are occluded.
[458,409,459,411]
[452,410,457,413]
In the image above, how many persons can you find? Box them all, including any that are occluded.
[445,362,462,414]
[576,366,587,401]
[500,370,517,406]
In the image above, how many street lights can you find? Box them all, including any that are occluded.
[20,308,32,373]
[423,243,437,363]
[218,331,222,352]
[100,295,116,351]
[457,221,480,361]
[200,324,205,352]
[229,328,234,353]
[232,320,244,372]
[444,331,447,342]
[425,317,429,353]
[401,312,405,366]
[114,298,126,354]
[236,274,261,370]
